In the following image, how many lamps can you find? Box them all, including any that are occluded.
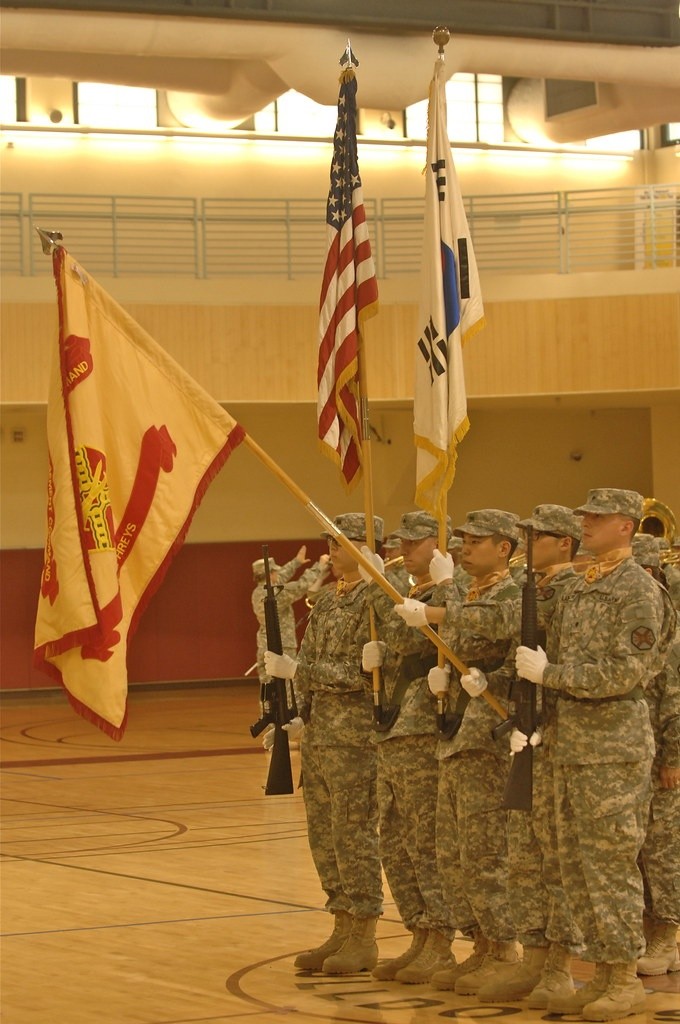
[381,112,395,129]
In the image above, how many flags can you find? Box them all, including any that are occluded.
[412,56,487,508]
[34,248,245,741]
[316,68,379,484]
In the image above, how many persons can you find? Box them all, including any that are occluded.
[357,502,680,1008]
[511,489,664,1020]
[252,546,329,718]
[263,513,384,974]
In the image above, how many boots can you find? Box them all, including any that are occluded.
[294,909,680,1021]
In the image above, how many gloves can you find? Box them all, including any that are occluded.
[308,571,330,592]
[262,717,305,752]
[460,667,488,698]
[514,644,548,684]
[394,597,429,627]
[264,650,299,679]
[427,663,451,696]
[428,549,455,585]
[509,726,541,756]
[357,546,384,583]
[361,640,386,673]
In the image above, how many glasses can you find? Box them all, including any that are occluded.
[523,527,568,541]
[327,537,343,548]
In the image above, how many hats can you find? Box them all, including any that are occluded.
[447,536,464,549]
[252,557,281,574]
[454,508,520,540]
[573,488,644,520]
[389,512,453,541]
[631,533,659,567]
[320,513,385,546]
[515,504,584,541]
[382,537,402,548]
[576,539,589,555]
[656,537,671,549]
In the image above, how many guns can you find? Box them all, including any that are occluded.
[498,522,548,814]
[250,542,296,796]
